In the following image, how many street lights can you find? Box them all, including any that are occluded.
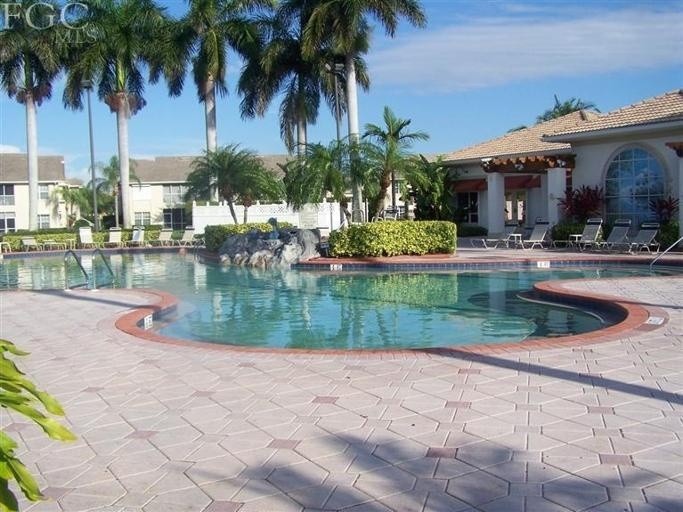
[78,76,98,234]
[323,60,348,233]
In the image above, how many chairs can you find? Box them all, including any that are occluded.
[470,217,661,255]
[0,225,198,253]
[350,207,400,223]
